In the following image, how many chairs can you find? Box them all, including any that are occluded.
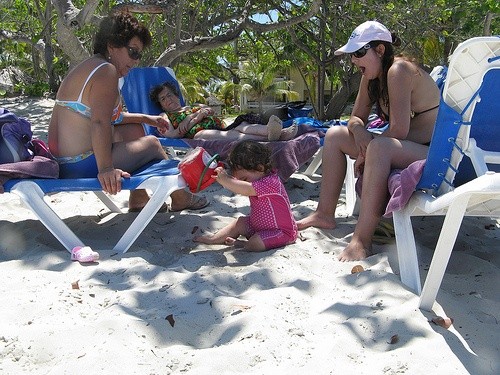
[118,67,325,184]
[0,106,187,255]
[345,36,500,311]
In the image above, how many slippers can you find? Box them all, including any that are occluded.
[169,193,210,214]
[129,202,167,212]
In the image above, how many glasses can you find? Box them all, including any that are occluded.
[350,44,371,58]
[127,46,143,60]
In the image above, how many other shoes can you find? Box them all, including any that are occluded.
[71,246,99,262]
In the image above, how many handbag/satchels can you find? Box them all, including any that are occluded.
[0,108,34,162]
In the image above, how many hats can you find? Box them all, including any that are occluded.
[346,21,391,56]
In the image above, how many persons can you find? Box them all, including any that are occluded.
[193,138,298,252]
[149,81,298,142]
[48,12,210,212]
[295,21,442,261]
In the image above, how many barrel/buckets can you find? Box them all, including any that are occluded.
[178,147,224,194]
